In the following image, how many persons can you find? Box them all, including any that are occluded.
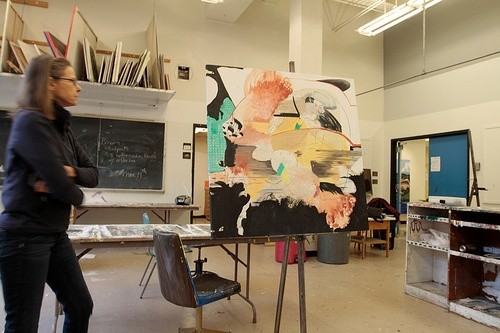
[0,54,99,333]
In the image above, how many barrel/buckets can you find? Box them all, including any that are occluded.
[317,233,349,264]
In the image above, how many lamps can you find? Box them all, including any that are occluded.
[357,0,442,36]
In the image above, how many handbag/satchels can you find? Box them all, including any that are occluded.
[367,206,385,223]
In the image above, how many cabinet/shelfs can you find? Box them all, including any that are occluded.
[351,217,397,261]
[405,202,500,329]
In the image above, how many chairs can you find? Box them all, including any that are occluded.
[153,229,242,333]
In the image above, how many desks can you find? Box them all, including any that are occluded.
[70,202,199,224]
[53,223,347,333]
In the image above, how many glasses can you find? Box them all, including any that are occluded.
[51,76,78,87]
